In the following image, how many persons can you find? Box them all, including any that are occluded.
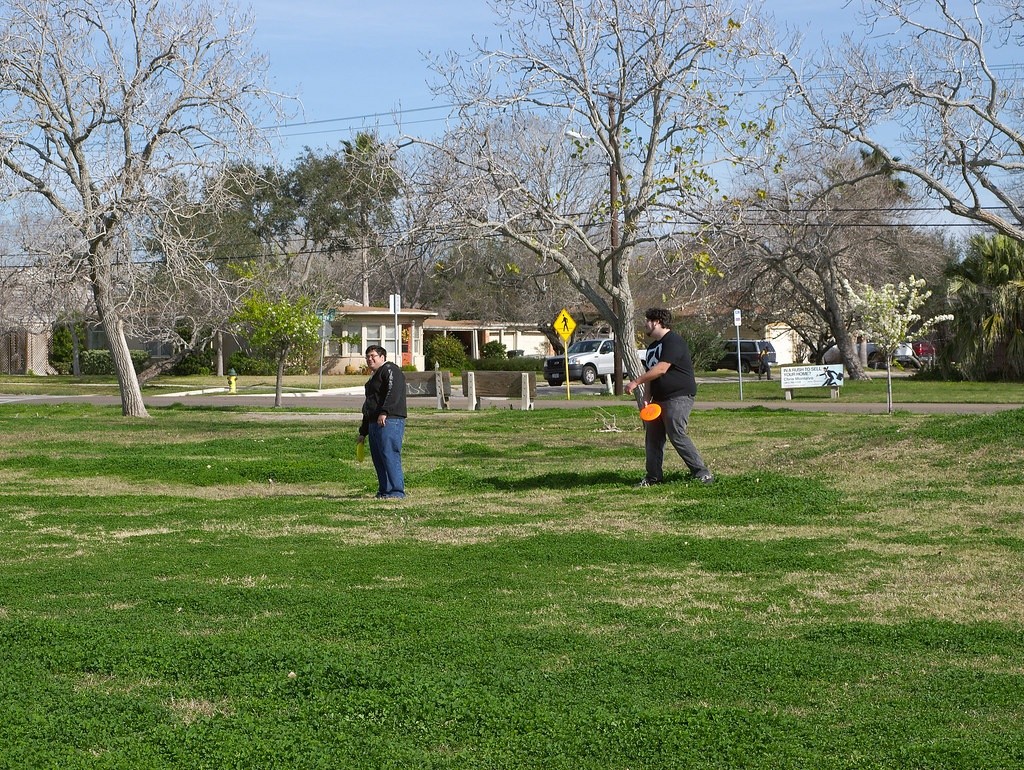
[625,308,712,487]
[357,345,407,499]
[759,346,773,380]
[817,367,838,386]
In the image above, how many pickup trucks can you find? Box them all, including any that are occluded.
[544,338,647,387]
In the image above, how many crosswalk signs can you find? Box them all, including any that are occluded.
[553,308,576,341]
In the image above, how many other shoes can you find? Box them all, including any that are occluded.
[768,378,773,380]
[759,375,761,380]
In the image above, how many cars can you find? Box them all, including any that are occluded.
[808,341,933,368]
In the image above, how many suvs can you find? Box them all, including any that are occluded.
[705,338,779,374]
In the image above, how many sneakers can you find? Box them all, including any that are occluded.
[693,474,712,485]
[638,479,662,488]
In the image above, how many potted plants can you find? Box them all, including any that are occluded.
[326,333,363,375]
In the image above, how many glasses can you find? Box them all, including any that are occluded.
[365,355,378,359]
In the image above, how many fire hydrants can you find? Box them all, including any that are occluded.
[227,368,239,394]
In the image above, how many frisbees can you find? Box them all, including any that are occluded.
[640,403,662,421]
[356,442,365,462]
